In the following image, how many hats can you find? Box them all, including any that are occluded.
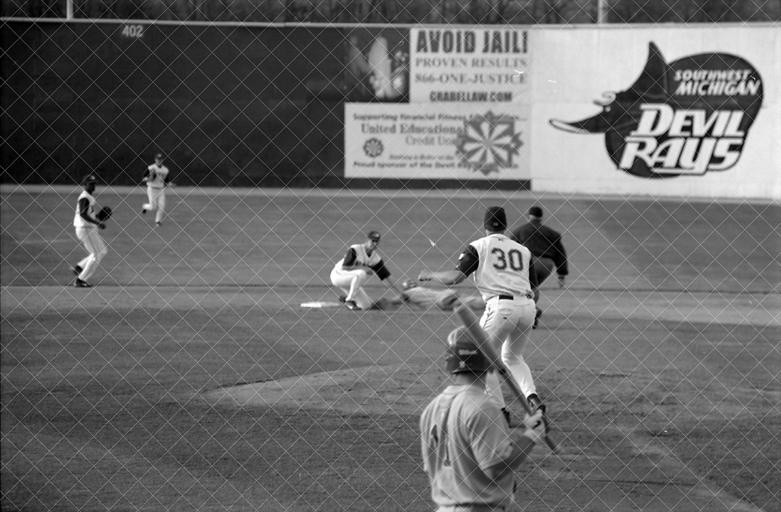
[154,153,163,160]
[529,206,544,218]
[483,206,508,232]
[368,230,381,242]
[83,175,97,183]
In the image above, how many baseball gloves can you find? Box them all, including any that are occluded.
[97,206,111,221]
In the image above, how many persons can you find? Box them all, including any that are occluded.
[375,274,489,312]
[416,324,547,512]
[140,153,176,226]
[418,204,552,436]
[511,205,570,331]
[69,173,107,289]
[330,230,406,310]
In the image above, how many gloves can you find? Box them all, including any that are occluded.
[521,408,549,446]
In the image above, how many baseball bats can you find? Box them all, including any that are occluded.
[454,303,562,456]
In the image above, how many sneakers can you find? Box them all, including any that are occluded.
[531,306,543,330]
[71,264,92,288]
[339,293,362,311]
[502,395,548,428]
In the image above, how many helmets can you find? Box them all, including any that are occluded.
[445,325,496,375]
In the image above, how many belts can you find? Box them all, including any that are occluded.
[499,293,533,301]
